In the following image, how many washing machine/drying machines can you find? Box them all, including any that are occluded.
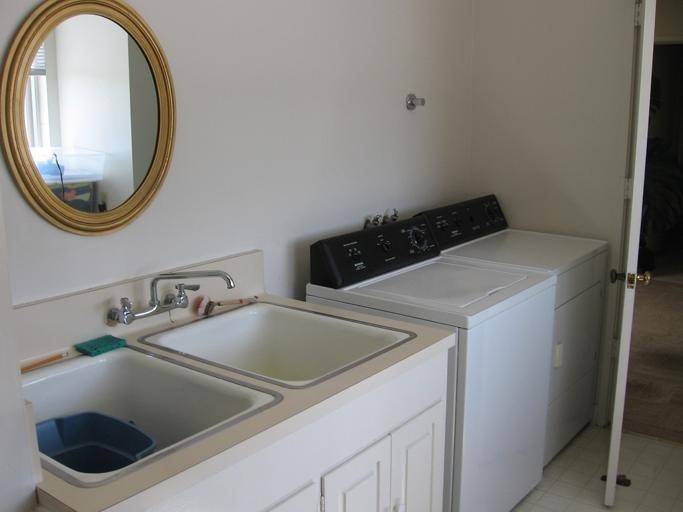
[307,214,557,512]
[423,192,610,467]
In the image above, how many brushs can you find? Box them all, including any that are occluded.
[195,296,256,315]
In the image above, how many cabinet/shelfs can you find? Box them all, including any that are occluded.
[269,399,445,512]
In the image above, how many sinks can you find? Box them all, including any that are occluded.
[138,297,418,390]
[19,347,274,492]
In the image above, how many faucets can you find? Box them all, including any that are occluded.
[107,267,235,326]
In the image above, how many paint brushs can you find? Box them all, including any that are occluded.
[21,353,69,373]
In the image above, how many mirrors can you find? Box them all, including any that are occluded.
[0,0,176,237]
